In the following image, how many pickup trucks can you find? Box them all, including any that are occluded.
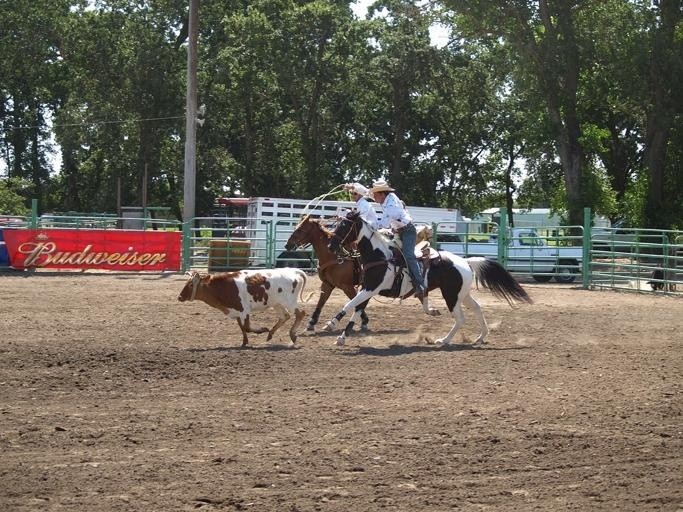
[435,225,584,284]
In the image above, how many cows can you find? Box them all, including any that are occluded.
[646,269,677,291]
[178,270,325,349]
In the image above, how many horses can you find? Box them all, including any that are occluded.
[285,216,443,336]
[322,207,535,348]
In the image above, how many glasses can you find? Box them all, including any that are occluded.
[350,192,358,195]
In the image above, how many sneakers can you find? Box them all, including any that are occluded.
[415,283,427,297]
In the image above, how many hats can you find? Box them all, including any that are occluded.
[349,186,367,196]
[370,180,396,193]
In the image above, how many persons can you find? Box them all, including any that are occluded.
[364,179,429,299]
[329,181,380,234]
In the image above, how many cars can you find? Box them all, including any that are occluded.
[592,217,640,259]
